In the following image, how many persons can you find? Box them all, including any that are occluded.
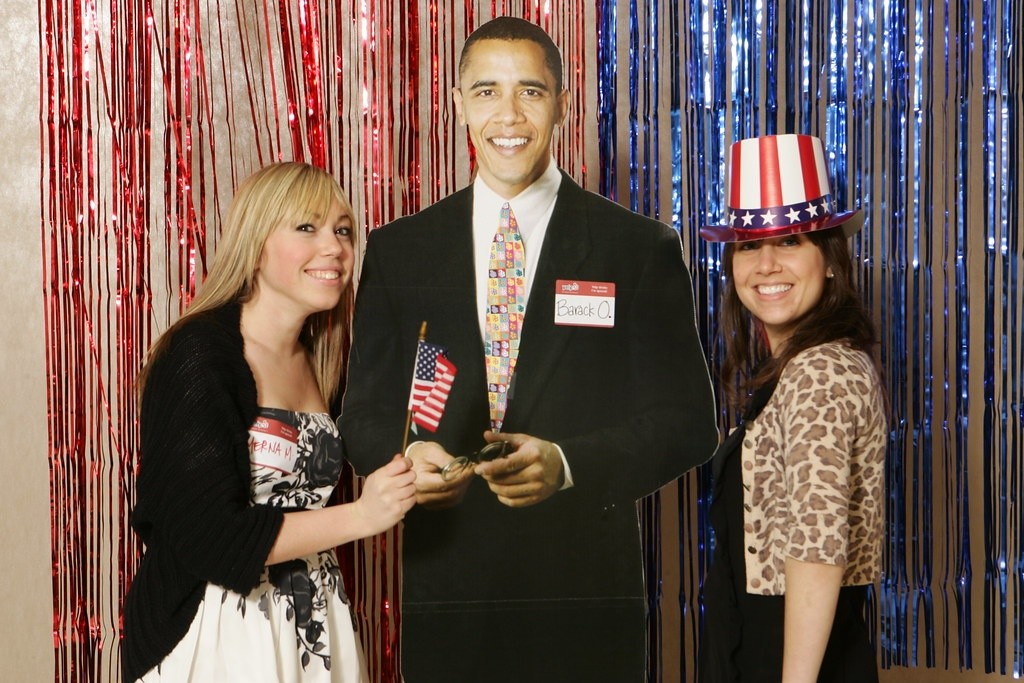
[336,16,721,682]
[699,134,889,683]
[120,160,415,682]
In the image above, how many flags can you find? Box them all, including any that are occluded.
[408,340,458,434]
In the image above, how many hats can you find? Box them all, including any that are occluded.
[700,134,861,243]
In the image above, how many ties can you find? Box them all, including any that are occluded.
[484,202,527,435]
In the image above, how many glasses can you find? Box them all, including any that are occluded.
[442,441,508,482]
